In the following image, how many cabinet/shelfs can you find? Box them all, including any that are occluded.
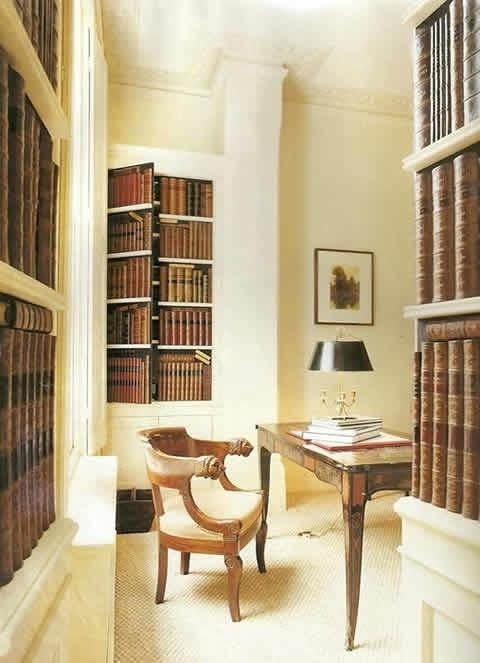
[0,2,223,655]
[404,1,480,658]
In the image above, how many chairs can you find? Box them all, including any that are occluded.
[140,427,268,622]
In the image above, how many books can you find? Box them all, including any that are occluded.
[284,410,413,452]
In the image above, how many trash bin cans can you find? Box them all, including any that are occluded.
[116,489,155,534]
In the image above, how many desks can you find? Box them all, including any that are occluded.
[250,422,412,650]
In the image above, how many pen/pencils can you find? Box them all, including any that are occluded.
[332,417,356,420]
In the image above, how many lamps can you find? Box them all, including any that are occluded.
[309,325,373,422]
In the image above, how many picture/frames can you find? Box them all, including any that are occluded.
[314,247,375,326]
